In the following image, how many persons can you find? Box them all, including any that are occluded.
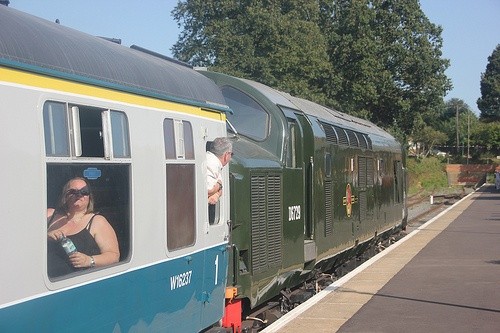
[494,169,500,190]
[206,137,233,205]
[47,176,121,280]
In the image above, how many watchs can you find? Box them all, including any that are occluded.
[91,256,95,267]
[219,182,223,190]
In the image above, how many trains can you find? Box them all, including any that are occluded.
[0,0,408,333]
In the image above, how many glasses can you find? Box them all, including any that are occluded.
[227,152,233,156]
[65,188,90,197]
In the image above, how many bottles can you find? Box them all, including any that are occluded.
[60,238,77,255]
